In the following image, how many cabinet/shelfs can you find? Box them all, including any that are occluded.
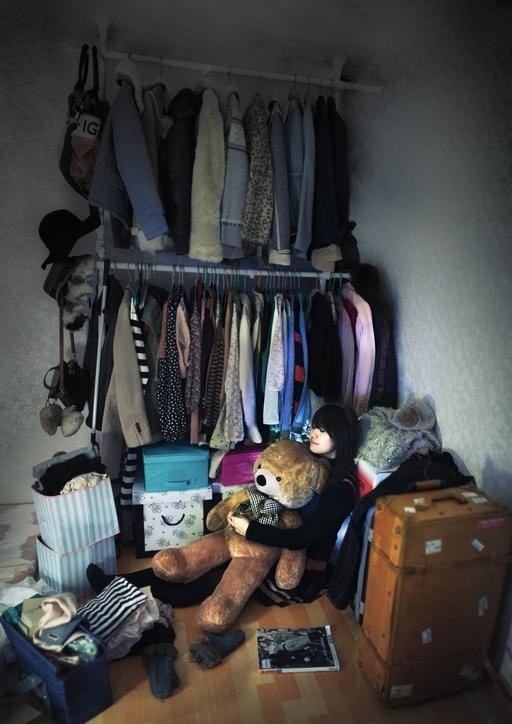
[348,503,375,624]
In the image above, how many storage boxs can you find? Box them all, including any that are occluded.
[218,448,260,484]
[33,536,118,593]
[141,444,209,490]
[217,483,249,499]
[131,482,213,552]
[30,476,120,551]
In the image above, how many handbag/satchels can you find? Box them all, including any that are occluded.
[44,360,87,412]
[59,45,109,199]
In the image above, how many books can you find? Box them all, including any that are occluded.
[256,624,341,674]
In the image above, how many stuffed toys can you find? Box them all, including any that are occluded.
[151,437,331,635]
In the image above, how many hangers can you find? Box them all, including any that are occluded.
[103,260,353,309]
[116,51,339,111]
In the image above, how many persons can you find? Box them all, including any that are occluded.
[258,631,327,655]
[85,402,360,613]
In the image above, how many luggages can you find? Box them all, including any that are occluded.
[362,486,511,709]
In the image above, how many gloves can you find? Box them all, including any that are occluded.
[141,642,179,700]
[189,628,245,669]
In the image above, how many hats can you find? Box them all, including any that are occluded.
[43,258,75,305]
[39,209,101,269]
